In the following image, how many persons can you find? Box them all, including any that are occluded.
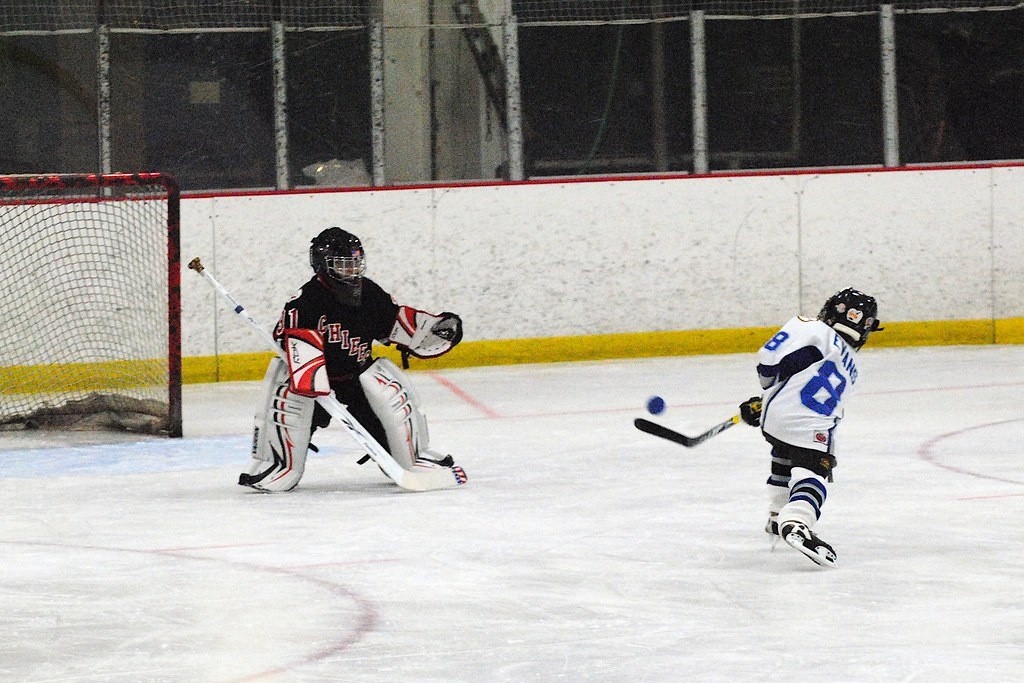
[237,226,462,494]
[740,287,879,562]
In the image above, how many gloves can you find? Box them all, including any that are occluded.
[739,397,762,427]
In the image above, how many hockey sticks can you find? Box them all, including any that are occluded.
[187,255,469,490]
[633,414,743,448]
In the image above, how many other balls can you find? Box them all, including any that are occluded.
[648,396,664,414]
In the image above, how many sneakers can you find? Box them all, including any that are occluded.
[766,510,781,552]
[778,514,838,569]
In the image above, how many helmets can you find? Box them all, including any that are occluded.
[310,227,364,290]
[817,287,884,352]
